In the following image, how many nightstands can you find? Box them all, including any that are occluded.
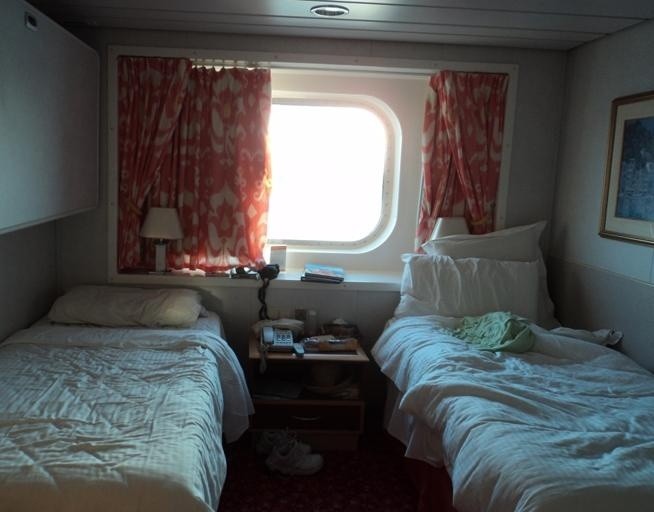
[243,330,370,454]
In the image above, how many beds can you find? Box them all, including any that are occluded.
[0,309,228,512]
[382,315,654,512]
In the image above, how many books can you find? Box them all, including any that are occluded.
[304,264,345,281]
[301,270,345,283]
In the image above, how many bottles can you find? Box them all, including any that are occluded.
[308,310,317,336]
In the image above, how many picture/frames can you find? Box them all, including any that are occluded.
[598,90,654,248]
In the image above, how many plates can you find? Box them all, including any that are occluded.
[307,385,341,394]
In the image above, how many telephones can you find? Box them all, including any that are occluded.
[259,326,293,352]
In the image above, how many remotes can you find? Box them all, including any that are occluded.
[294,342,304,356]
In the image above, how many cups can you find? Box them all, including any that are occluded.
[312,363,337,389]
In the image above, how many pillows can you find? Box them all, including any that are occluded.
[395,215,556,325]
[48,280,201,327]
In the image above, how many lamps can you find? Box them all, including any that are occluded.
[138,207,186,277]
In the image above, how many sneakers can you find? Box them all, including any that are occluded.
[262,430,323,475]
